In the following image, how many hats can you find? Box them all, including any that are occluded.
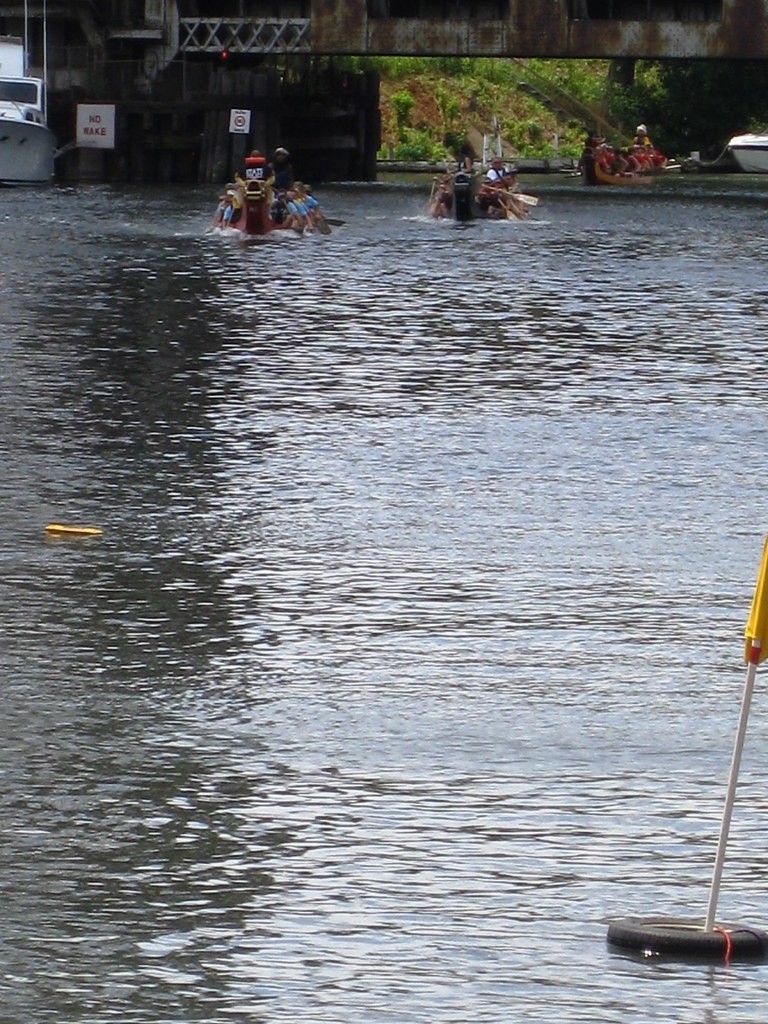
[294,182,303,189]
[225,182,236,191]
[276,148,289,155]
[252,150,260,156]
[636,125,647,134]
[491,156,503,163]
[304,184,311,191]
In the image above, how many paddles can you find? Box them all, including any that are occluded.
[317,218,346,234]
[425,175,437,210]
[482,183,540,208]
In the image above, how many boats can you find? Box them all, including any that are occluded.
[0,76,56,184]
[576,122,680,188]
[727,119,768,175]
[212,145,332,240]
[425,116,528,228]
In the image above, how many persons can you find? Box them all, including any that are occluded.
[205,148,321,234]
[432,157,526,218]
[586,125,668,178]
[547,128,558,149]
